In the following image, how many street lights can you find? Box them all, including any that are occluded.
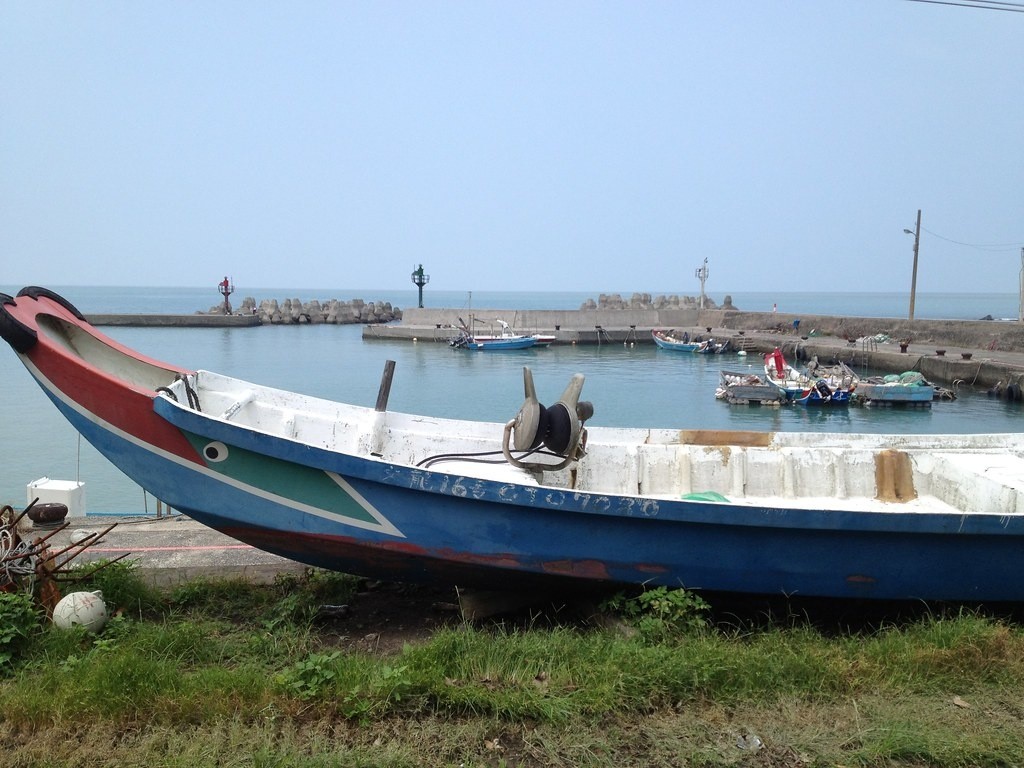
[903,209,922,318]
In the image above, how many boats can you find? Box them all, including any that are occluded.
[469,318,558,347]
[650,329,732,354]
[804,353,936,407]
[800,353,855,403]
[717,368,782,408]
[0,285,1024,622]
[448,291,539,353]
[759,347,833,406]
